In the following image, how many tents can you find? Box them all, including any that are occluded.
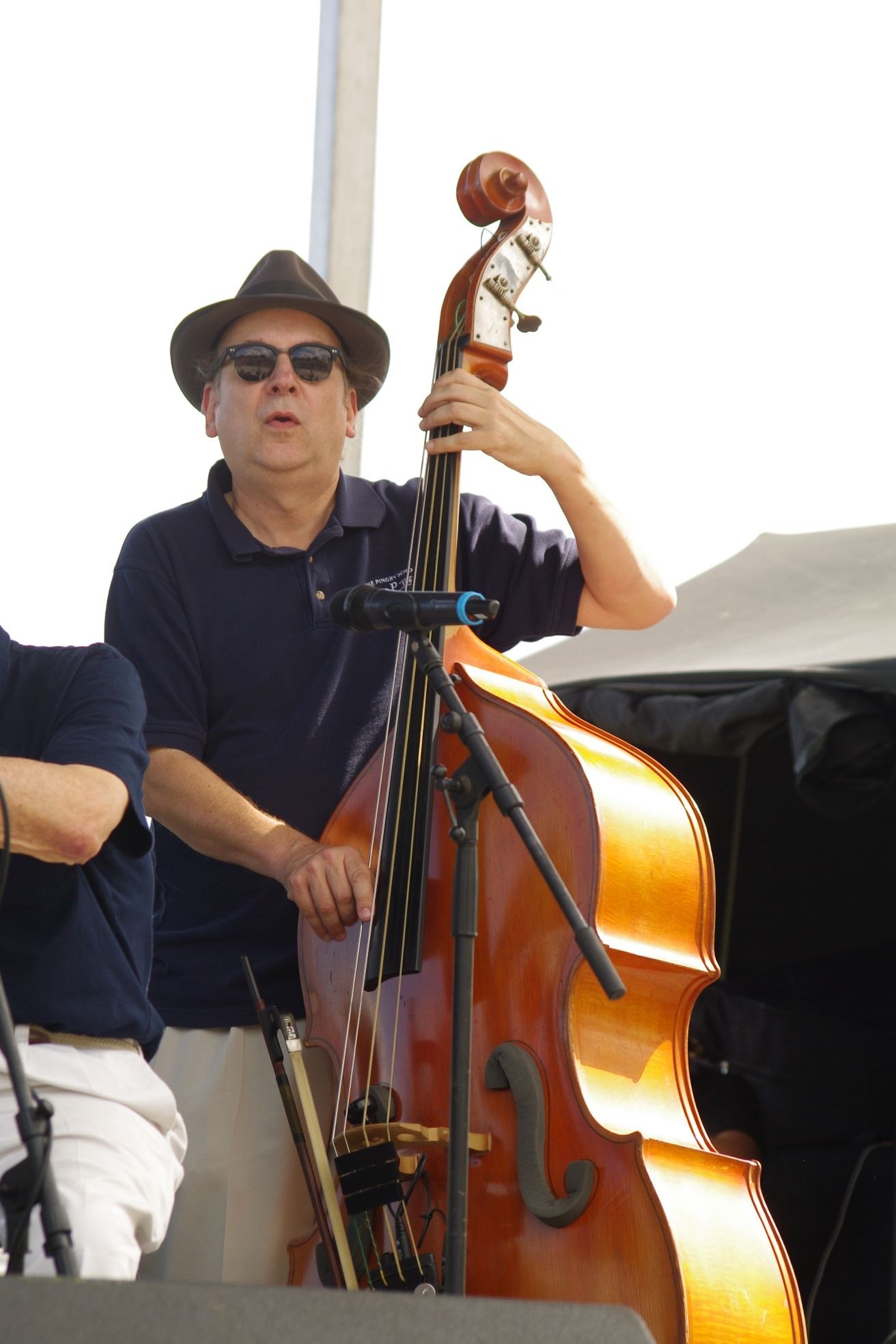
[512,522,896,1342]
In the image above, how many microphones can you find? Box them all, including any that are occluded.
[330,584,501,634]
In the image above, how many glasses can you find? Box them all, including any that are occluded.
[209,341,351,382]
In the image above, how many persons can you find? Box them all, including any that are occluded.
[1,632,189,1281]
[101,277,678,1287]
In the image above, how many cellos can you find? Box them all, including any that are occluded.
[294,151,810,1344]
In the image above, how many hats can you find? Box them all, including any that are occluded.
[170,250,391,416]
